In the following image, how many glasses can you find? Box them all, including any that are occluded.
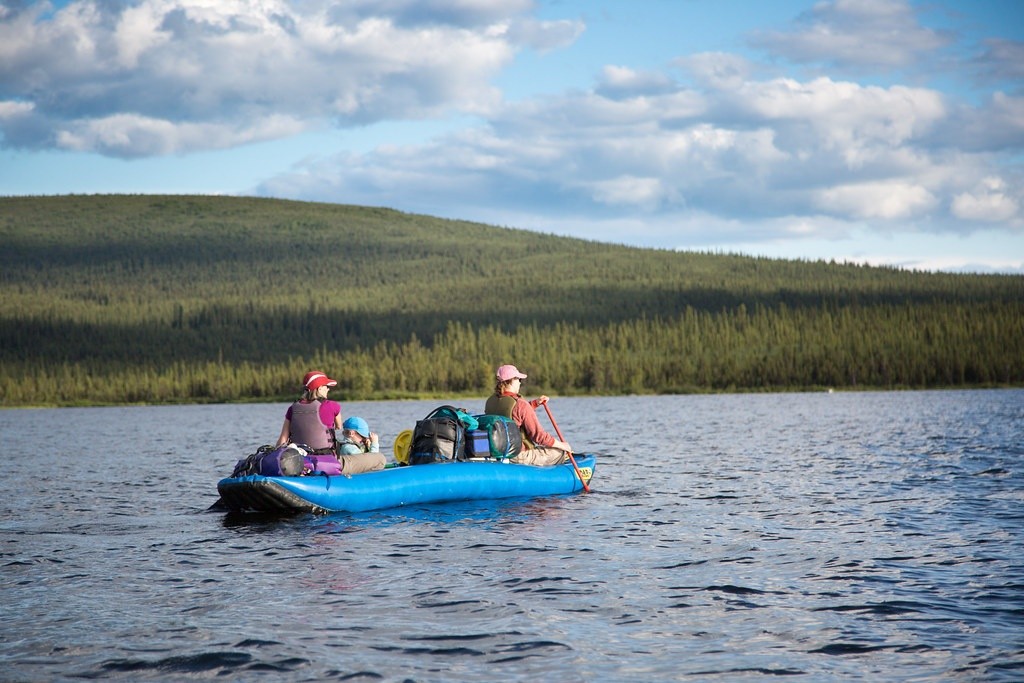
[322,385,330,389]
[512,377,522,383]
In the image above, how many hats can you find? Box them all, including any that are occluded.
[343,417,370,438]
[303,371,337,390]
[496,365,528,382]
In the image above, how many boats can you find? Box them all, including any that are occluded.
[216,447,597,515]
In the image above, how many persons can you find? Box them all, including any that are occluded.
[337,417,379,455]
[484,364,572,466]
[276,371,386,474]
